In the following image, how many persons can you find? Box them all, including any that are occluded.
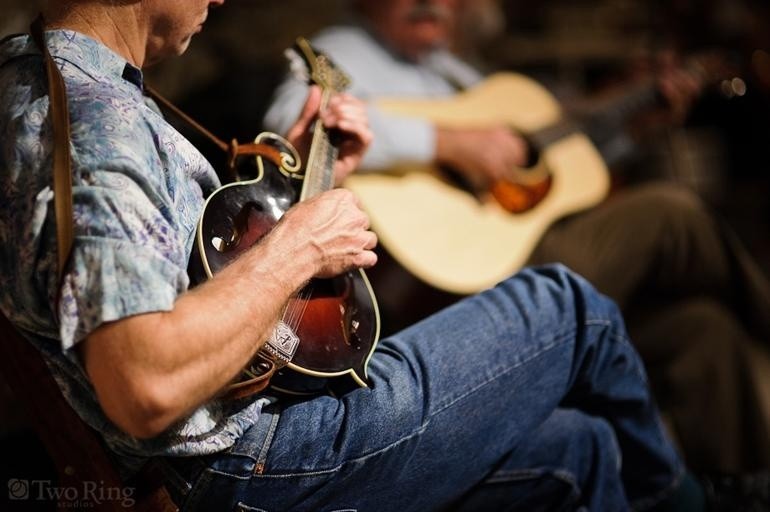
[259,0,769,511]
[0,0,706,512]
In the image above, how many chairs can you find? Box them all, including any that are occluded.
[0,312,182,512]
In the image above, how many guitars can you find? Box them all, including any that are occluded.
[187,34,383,396]
[339,49,748,309]
